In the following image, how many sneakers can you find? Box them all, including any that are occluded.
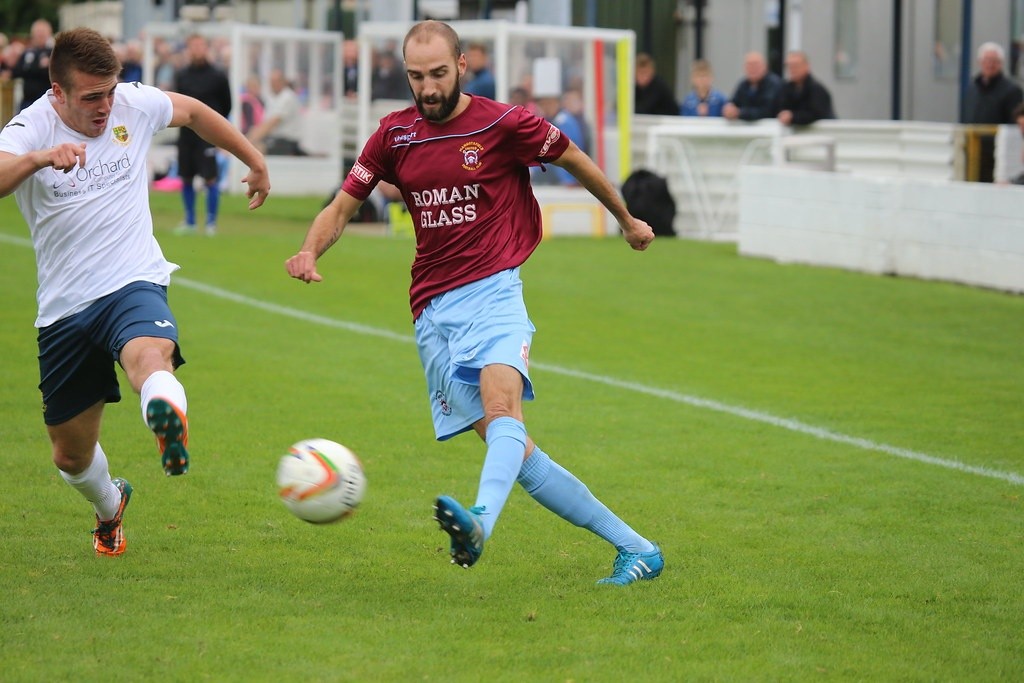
[432,494,489,569]
[90,476,134,560]
[143,394,190,476]
[596,541,665,585]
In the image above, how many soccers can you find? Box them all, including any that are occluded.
[276,437,367,526]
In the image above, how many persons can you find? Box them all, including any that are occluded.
[720,49,782,122]
[636,53,680,115]
[0,22,303,196]
[284,22,665,587]
[958,43,1024,183]
[327,42,590,223]
[169,33,232,227]
[0,28,273,555]
[682,58,726,116]
[768,51,832,126]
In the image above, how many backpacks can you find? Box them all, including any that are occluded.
[622,168,676,237]
